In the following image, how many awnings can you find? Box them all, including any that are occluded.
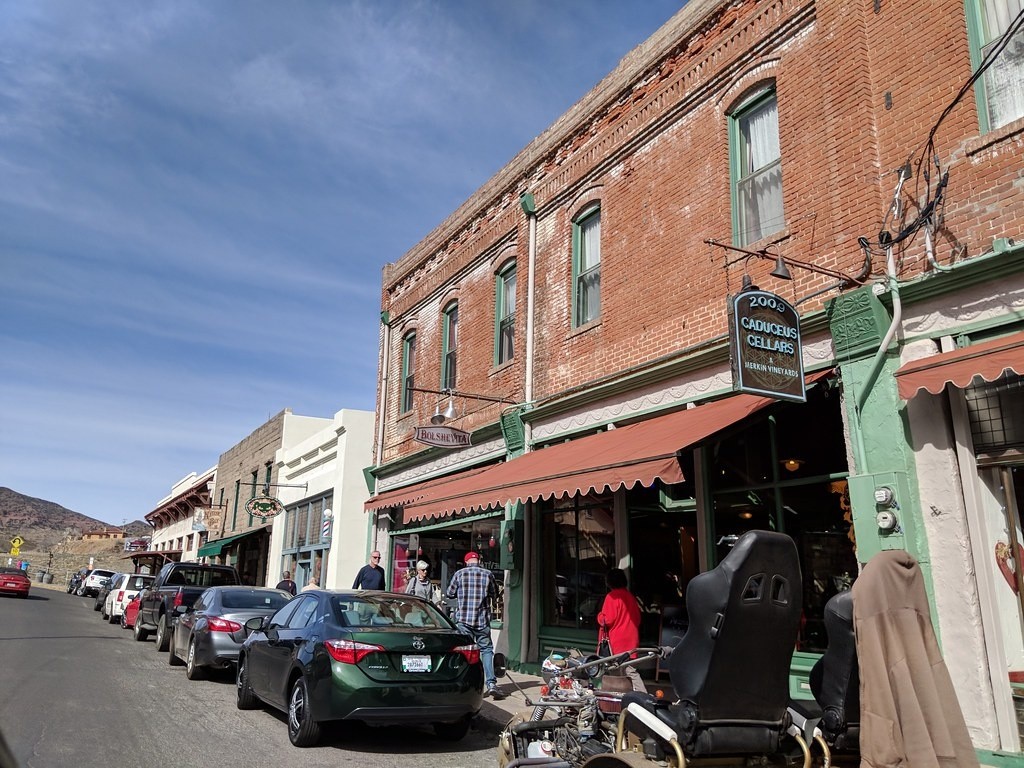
[364,368,833,524]
[197,525,272,557]
[122,550,182,559]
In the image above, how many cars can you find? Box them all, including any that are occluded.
[93,573,123,611]
[77,568,117,596]
[169,585,294,681]
[121,587,183,628]
[0,567,31,599]
[67,569,93,594]
[141,534,151,541]
[236,589,485,747]
[127,539,148,551]
[101,573,156,624]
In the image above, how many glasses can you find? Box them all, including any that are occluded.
[421,569,427,572]
[372,556,381,559]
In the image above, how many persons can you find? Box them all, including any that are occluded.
[596,569,638,668]
[446,553,506,698]
[16,559,29,570]
[276,571,297,596]
[301,577,321,611]
[405,560,432,601]
[349,551,385,625]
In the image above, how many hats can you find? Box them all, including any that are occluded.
[244,571,251,575]
[464,552,479,561]
[416,560,429,570]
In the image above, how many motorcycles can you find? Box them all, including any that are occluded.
[492,530,860,768]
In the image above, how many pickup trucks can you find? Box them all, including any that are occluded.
[133,562,242,650]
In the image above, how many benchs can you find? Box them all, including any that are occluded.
[344,611,423,626]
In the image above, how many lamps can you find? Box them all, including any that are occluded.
[742,244,791,292]
[432,387,458,425]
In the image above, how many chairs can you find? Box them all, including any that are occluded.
[784,550,926,768]
[621,528,804,768]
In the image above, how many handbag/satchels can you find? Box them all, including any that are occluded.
[596,618,613,658]
[410,577,417,595]
